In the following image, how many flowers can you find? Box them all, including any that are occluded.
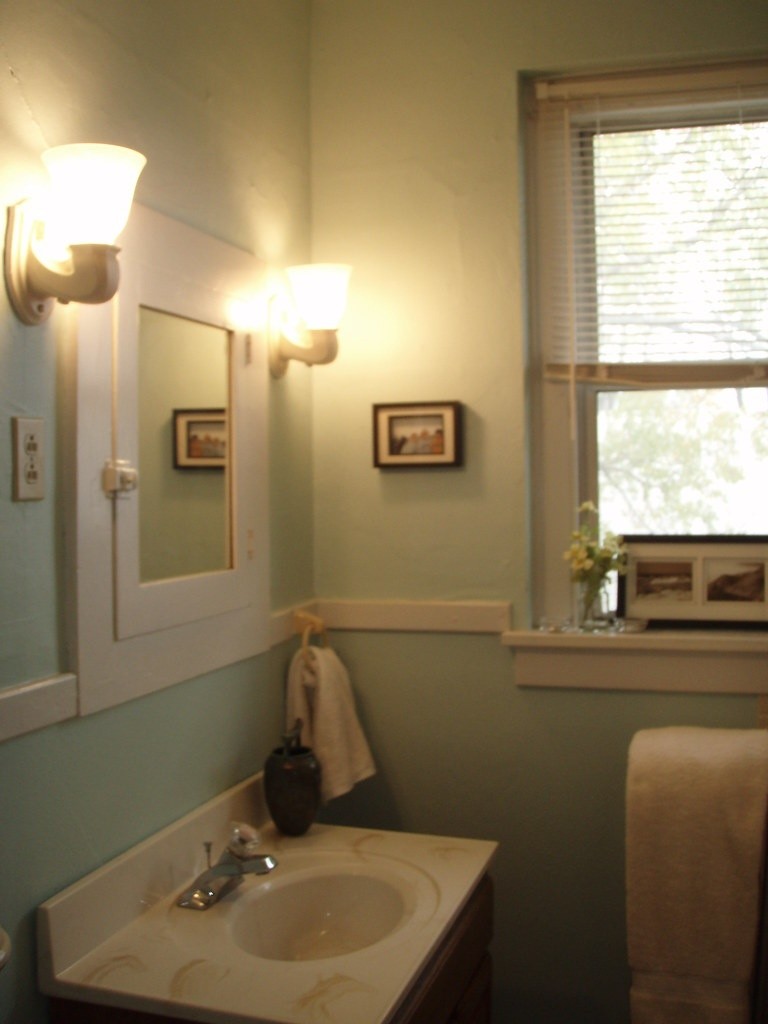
[562,501,630,622]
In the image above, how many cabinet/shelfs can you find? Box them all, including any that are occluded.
[401,867,497,1024]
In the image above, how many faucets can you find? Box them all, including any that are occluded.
[175,823,279,911]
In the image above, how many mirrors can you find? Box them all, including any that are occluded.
[138,304,232,584]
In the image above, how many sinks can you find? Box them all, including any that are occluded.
[215,847,442,976]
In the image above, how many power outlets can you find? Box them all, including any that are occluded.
[13,416,47,502]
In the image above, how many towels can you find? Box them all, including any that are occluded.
[285,647,377,803]
[623,724,768,1024]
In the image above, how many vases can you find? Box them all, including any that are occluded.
[574,578,609,630]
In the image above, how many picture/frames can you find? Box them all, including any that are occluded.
[619,535,768,630]
[172,409,229,469]
[373,401,464,468]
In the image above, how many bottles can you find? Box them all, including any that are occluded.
[264,717,322,839]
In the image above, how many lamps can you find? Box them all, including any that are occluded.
[5,143,148,327]
[267,261,354,378]
